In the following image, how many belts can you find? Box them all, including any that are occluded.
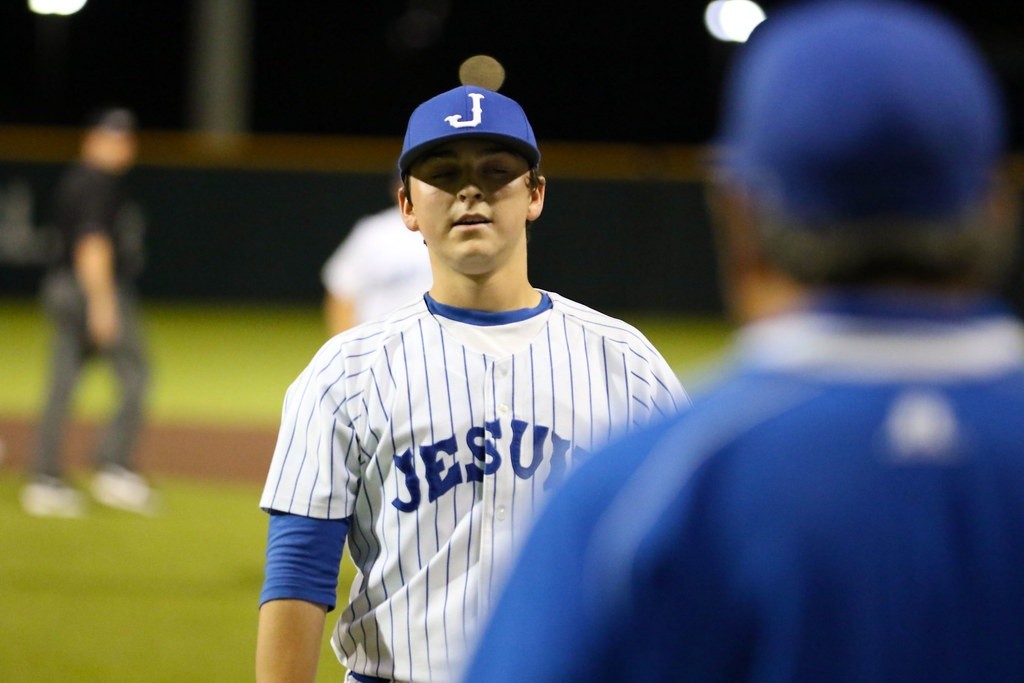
[349,671,393,683]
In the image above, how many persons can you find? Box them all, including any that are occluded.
[459,0,1024,683]
[257,85,694,683]
[20,108,151,519]
[322,199,433,339]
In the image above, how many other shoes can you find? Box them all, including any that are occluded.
[23,482,84,520]
[91,468,150,509]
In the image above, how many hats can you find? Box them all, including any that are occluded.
[717,5,1001,225]
[394,87,541,182]
